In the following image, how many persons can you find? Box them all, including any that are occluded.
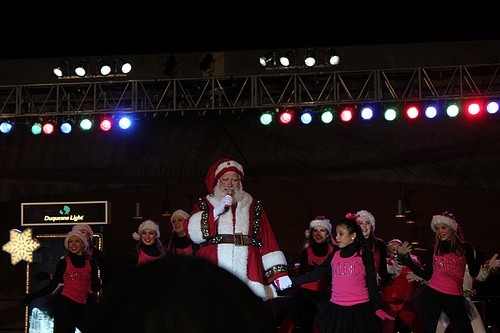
[19,194,500,333]
[273,209,411,333]
[115,218,171,285]
[162,207,200,261]
[182,156,293,302]
[392,208,500,333]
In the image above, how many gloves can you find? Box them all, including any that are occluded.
[274,275,292,291]
[214,194,233,218]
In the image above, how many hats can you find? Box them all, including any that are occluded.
[305,215,332,238]
[431,211,458,233]
[131,220,160,240]
[171,209,189,223]
[72,224,93,243]
[390,238,402,244]
[356,210,375,232]
[64,230,88,250]
[205,157,244,195]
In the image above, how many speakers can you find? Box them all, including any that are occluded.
[24,233,104,333]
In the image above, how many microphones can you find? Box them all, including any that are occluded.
[224,190,233,211]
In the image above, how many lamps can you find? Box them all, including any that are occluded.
[132,184,143,220]
[51,56,132,77]
[394,183,427,252]
[24,117,43,135]
[161,188,172,217]
[199,54,213,71]
[257,48,344,66]
[161,53,180,76]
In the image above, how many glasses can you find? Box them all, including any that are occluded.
[81,231,87,236]
